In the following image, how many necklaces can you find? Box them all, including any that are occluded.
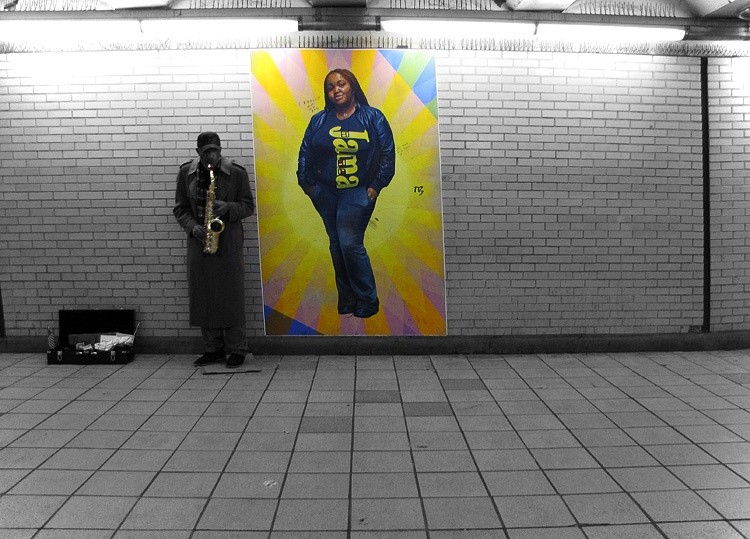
[343,113,347,116]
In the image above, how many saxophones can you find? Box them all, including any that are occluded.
[202,164,225,253]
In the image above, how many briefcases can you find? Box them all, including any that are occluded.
[48,308,137,365]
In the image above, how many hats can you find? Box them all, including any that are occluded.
[198,132,220,152]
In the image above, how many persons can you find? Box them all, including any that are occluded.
[296,68,396,320]
[172,131,255,368]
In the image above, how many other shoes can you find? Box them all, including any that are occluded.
[227,353,244,368]
[338,304,354,314]
[354,308,374,317]
[195,353,224,365]
